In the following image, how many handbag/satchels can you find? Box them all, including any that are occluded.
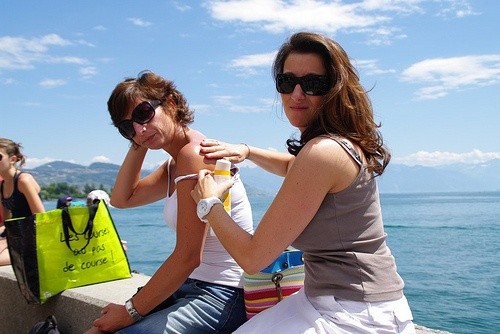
[242,250,306,321]
[4,198,132,306]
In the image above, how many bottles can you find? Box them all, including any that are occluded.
[210,159,232,237]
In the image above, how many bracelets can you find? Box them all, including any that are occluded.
[241,143,250,159]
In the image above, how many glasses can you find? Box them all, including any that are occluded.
[275,72,340,96]
[116,99,163,139]
[0,154,13,161]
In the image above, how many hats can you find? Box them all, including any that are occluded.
[87,190,111,205]
[60,196,72,205]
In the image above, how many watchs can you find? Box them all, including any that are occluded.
[125,299,145,322]
[197,197,223,222]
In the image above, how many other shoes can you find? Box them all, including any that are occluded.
[39,314,60,334]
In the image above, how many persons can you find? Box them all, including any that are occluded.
[199,31,416,334]
[57,196,72,208]
[84,70,254,334]
[0,138,45,266]
